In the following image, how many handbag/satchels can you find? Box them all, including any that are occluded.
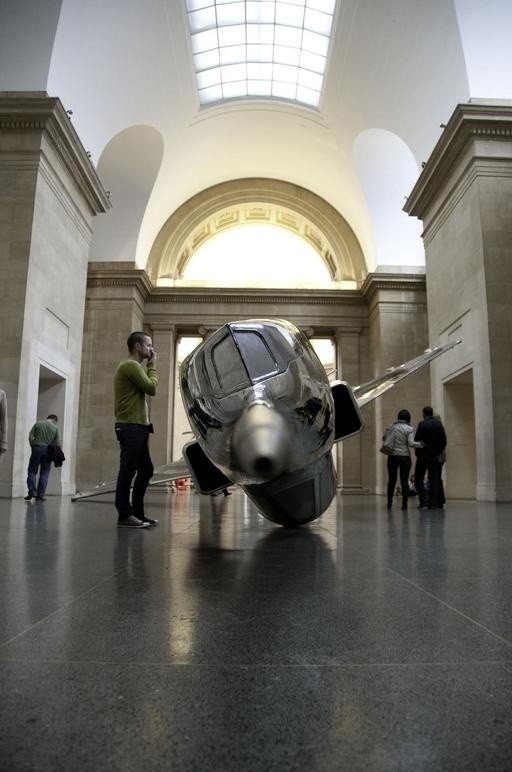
[377,423,397,456]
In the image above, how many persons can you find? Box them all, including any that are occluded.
[25,414,60,502]
[383,407,426,509]
[414,407,448,510]
[404,473,419,496]
[210,488,233,497]
[112,331,160,528]
[433,414,447,509]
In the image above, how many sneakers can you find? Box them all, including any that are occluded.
[117,515,159,529]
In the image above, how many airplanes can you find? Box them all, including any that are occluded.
[69,317,464,529]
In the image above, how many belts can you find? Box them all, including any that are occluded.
[114,427,123,431]
[35,444,46,448]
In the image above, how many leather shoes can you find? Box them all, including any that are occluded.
[24,493,47,502]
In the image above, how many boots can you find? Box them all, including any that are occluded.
[387,482,395,509]
[400,485,409,510]
